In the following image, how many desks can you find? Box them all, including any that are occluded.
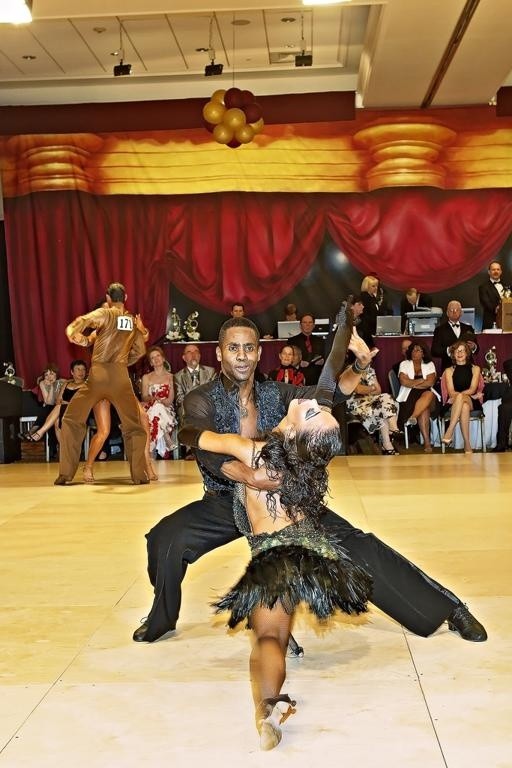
[159,334,512,380]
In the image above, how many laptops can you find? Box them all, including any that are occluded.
[458,307,476,328]
[277,320,303,340]
[374,315,403,337]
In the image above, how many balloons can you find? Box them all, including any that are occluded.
[200,87,265,149]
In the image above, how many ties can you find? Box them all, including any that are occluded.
[193,370,199,385]
[305,335,312,354]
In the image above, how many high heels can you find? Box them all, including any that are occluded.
[255,694,297,750]
[380,418,472,454]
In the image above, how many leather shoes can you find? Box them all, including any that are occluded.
[135,476,149,484]
[26,432,41,442]
[166,444,178,452]
[54,475,72,484]
[448,604,488,641]
[146,472,158,480]
[134,619,176,642]
[82,464,94,482]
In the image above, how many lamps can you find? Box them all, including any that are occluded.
[295,16,312,67]
[204,13,223,75]
[111,20,131,75]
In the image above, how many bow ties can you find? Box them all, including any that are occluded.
[494,281,500,284]
[452,324,459,327]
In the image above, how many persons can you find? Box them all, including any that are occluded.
[133,316,488,643]
[479,261,511,330]
[138,344,217,462]
[231,303,244,317]
[266,276,484,456]
[176,294,364,753]
[52,282,158,485]
[20,359,86,448]
[490,359,512,452]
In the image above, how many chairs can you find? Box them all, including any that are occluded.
[441,400,486,455]
[19,378,357,460]
[389,372,442,451]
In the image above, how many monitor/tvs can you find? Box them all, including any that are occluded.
[404,307,443,337]
[311,318,330,339]
